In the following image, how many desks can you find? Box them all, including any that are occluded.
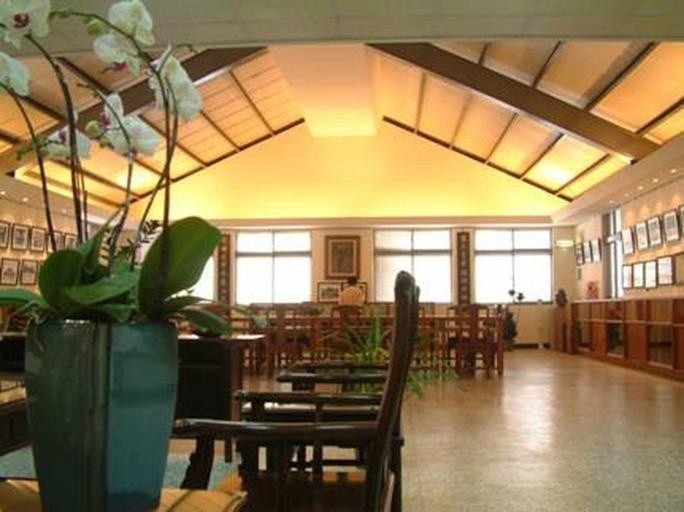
[1,476,250,512]
[1,330,266,440]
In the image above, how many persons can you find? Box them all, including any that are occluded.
[338,274,365,307]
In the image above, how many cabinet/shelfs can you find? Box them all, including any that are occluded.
[570,299,684,382]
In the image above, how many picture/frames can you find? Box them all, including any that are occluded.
[324,234,360,281]
[0,220,79,287]
[573,203,684,288]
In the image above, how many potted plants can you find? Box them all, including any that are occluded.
[493,289,525,351]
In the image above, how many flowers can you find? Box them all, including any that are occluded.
[0,0,268,340]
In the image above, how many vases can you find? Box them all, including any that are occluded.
[24,321,179,512]
